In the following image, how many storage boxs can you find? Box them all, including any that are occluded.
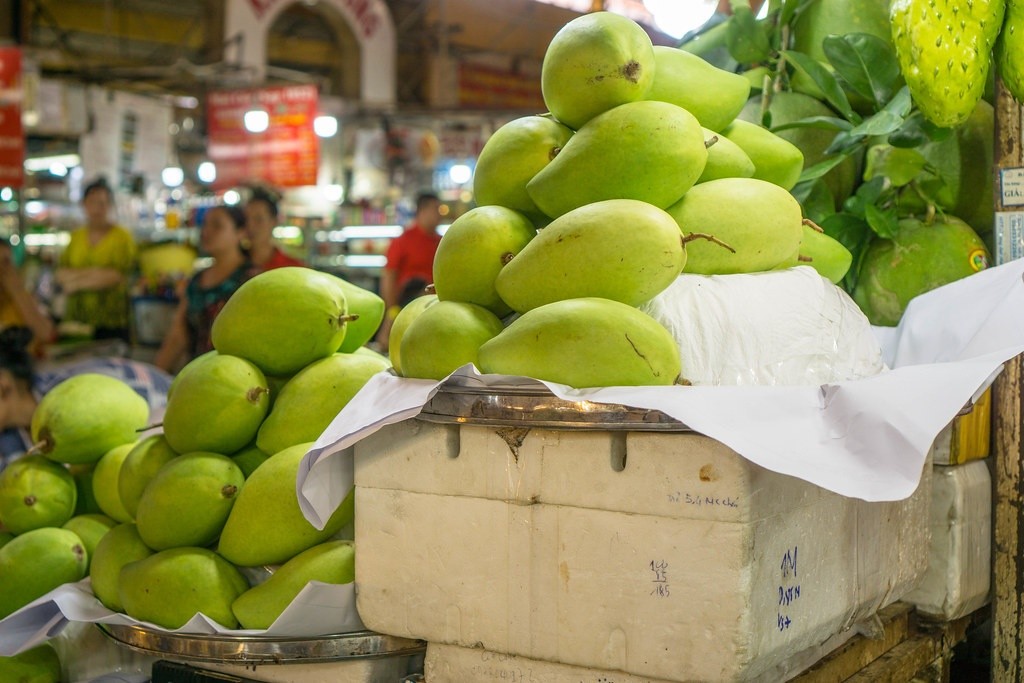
[354,426,931,683]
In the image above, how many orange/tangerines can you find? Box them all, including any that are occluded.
[684,0,999,326]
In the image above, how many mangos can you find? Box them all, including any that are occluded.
[386,12,856,388]
[0,266,393,683]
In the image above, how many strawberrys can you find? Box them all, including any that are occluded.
[885,0,1024,130]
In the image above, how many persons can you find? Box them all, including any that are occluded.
[153,187,308,374]
[0,236,51,473]
[58,178,136,346]
[377,192,443,353]
